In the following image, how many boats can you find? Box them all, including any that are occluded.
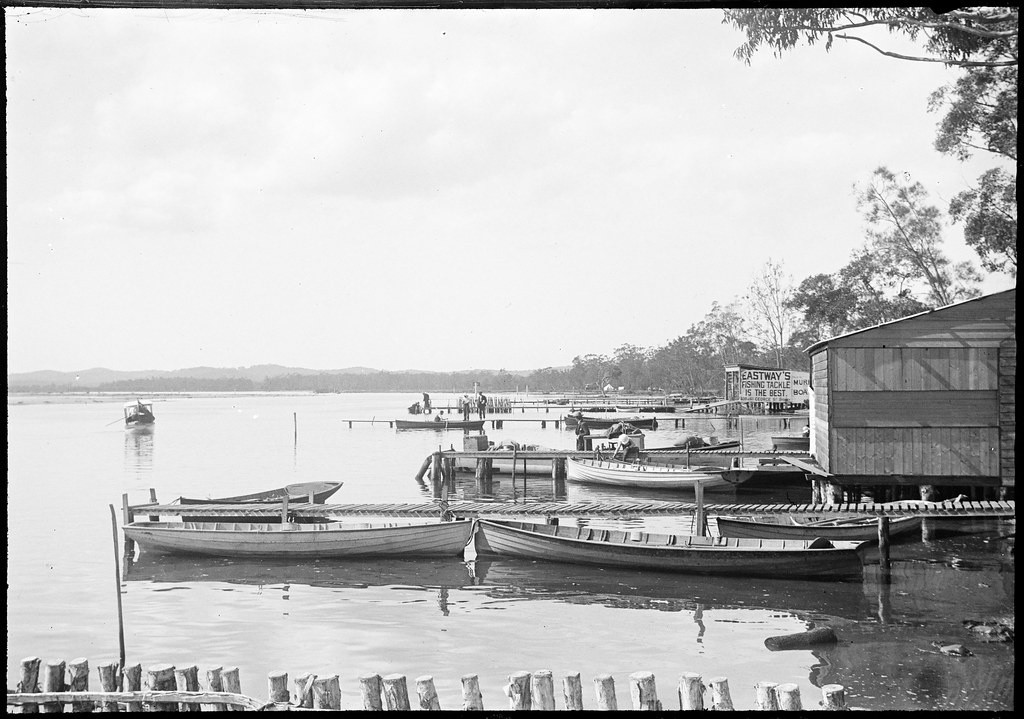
[394,418,486,430]
[565,452,734,488]
[563,413,658,430]
[125,398,155,425]
[121,516,474,558]
[123,552,472,588]
[176,478,342,504]
[717,514,924,540]
[466,554,869,619]
[474,518,871,582]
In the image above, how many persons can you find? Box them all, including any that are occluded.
[461,394,470,421]
[434,410,448,422]
[573,411,592,450]
[610,433,641,463]
[422,392,431,408]
[477,391,487,420]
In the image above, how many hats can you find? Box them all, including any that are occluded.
[572,411,584,418]
[619,434,629,445]
